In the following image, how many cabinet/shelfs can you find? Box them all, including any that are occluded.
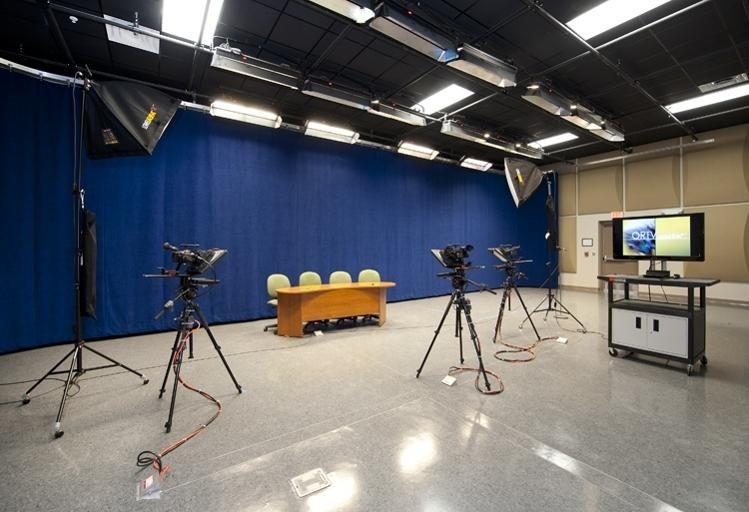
[596,273,721,377]
[264,268,381,335]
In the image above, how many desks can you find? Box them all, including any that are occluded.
[275,281,396,337]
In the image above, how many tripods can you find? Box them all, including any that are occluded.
[159,308,242,432]
[417,291,490,391]
[19,78,150,438]
[493,283,541,343]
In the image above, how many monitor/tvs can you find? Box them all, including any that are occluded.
[612,211,705,278]
[519,174,586,334]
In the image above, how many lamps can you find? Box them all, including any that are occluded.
[504,157,587,334]
[21,72,181,438]
